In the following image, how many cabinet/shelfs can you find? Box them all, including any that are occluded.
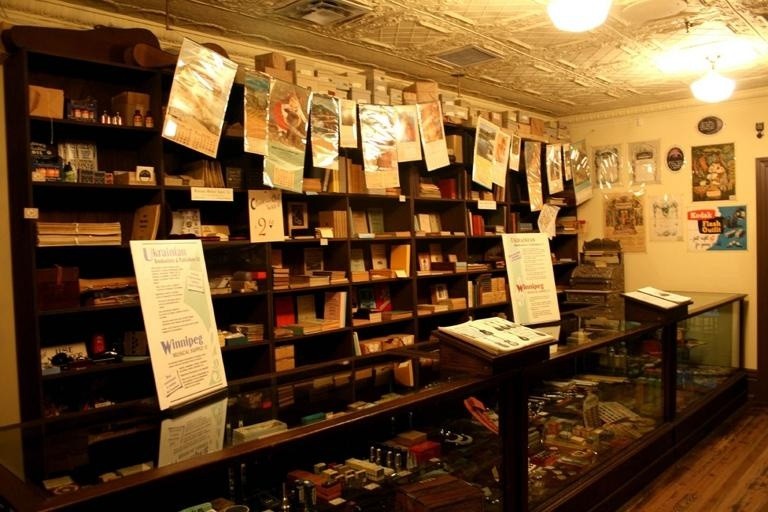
[14,47,272,421]
[401,309,671,510]
[416,117,512,349]
[271,93,417,373]
[1,347,520,511]
[511,138,577,311]
[588,290,749,464]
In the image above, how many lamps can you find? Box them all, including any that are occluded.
[690,53,736,104]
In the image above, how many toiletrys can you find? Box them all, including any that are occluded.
[369,446,402,470]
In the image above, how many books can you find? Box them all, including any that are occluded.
[467,209,504,236]
[557,216,577,234]
[352,287,413,326]
[40,342,88,377]
[468,258,504,272]
[468,184,505,202]
[414,214,464,236]
[228,350,442,406]
[36,204,162,247]
[419,176,463,199]
[218,324,264,345]
[416,244,467,276]
[195,159,225,187]
[417,284,467,316]
[315,211,347,238]
[546,195,567,206]
[271,250,349,290]
[302,157,401,195]
[353,207,410,237]
[273,292,347,339]
[352,244,410,282]
[468,277,506,308]
[510,212,532,233]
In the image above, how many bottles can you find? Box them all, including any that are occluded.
[607,342,662,377]
[278,478,318,511]
[623,378,663,418]
[68,96,153,127]
[367,444,402,471]
[676,367,690,391]
[92,335,107,354]
